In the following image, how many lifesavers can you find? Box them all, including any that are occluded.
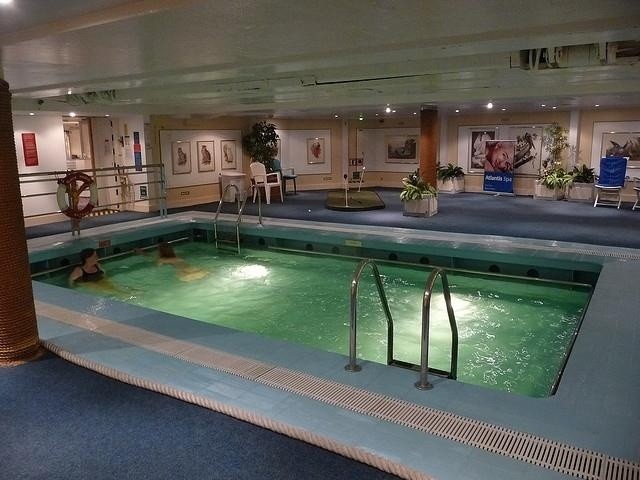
[56,173,98,218]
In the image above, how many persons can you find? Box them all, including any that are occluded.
[484,140,514,174]
[514,132,538,161]
[472,131,492,168]
[134,241,183,267]
[69,247,106,288]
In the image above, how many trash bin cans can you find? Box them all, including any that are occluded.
[221,171,247,203]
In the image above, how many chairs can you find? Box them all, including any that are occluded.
[248,159,298,205]
[592,158,640,211]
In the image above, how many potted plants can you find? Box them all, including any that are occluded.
[532,121,594,202]
[436,160,464,194]
[241,121,284,199]
[400,168,439,216]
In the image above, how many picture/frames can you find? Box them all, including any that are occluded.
[602,131,640,168]
[171,140,237,175]
[468,127,543,174]
[307,138,325,164]
[384,134,420,165]
